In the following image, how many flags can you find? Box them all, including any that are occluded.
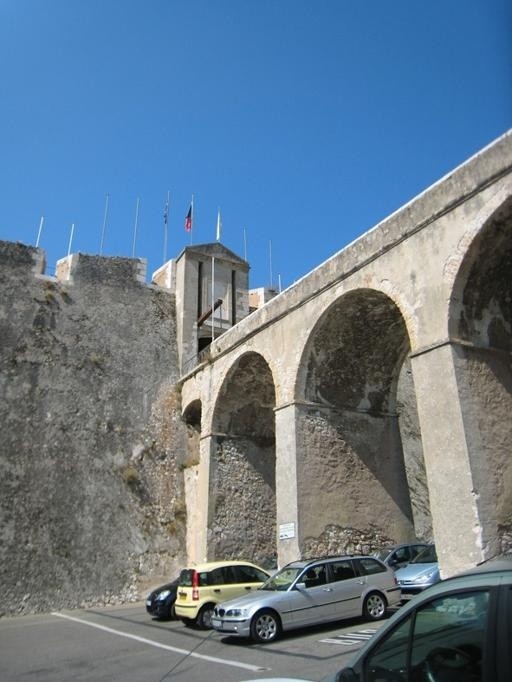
[184,203,192,232]
[216,210,221,241]
[163,202,169,225]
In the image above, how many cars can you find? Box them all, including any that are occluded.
[321,553,512,682]
[372,542,440,600]
[145,576,181,620]
[211,553,402,643]
[174,560,292,630]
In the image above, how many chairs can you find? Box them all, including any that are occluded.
[305,569,321,587]
